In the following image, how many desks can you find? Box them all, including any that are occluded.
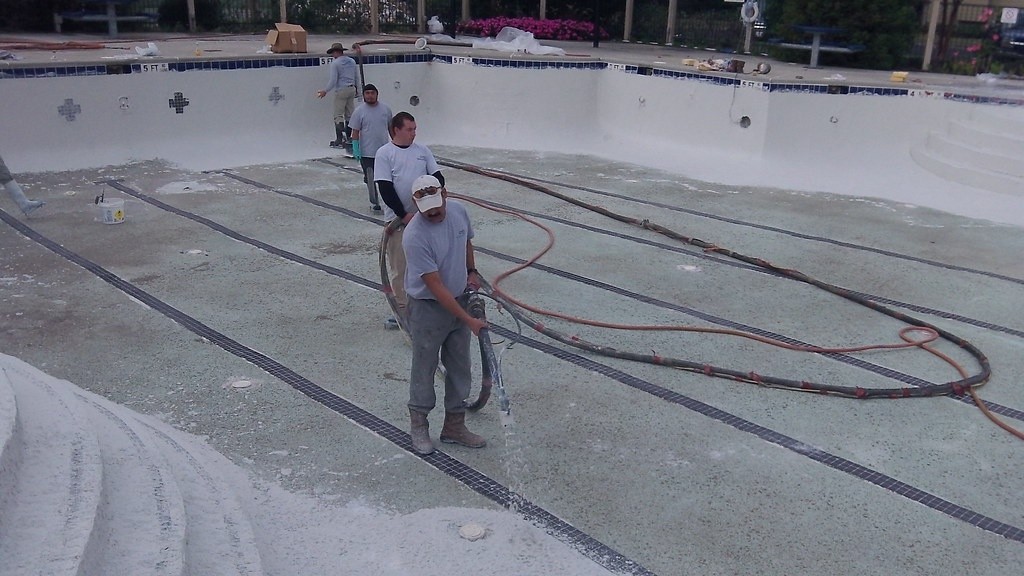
[792,25,850,66]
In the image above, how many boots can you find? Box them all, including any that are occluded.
[329,121,353,149]
[440,409,486,448]
[409,407,435,455]
[3,179,42,214]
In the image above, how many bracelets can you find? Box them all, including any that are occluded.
[467,269,477,274]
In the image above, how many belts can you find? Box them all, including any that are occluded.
[348,85,354,87]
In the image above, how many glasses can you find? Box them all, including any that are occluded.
[413,186,443,199]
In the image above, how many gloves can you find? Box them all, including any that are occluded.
[352,140,361,161]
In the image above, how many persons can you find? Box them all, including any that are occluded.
[402,176,487,455]
[317,42,362,146]
[373,110,446,329]
[347,84,393,210]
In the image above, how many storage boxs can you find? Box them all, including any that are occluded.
[265,23,307,53]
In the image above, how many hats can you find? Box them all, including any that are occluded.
[363,84,379,95]
[412,174,443,213]
[327,43,348,54]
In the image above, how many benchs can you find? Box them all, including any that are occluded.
[767,38,867,53]
[60,12,161,23]
